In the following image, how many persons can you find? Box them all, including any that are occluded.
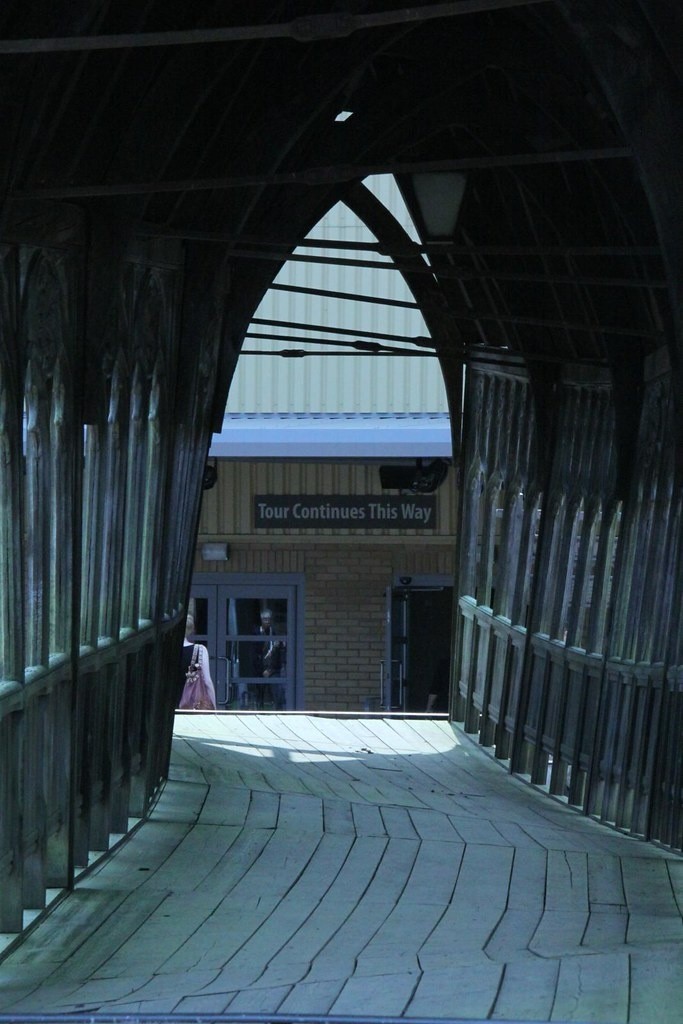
[426,641,451,713]
[178,615,216,710]
[251,611,286,710]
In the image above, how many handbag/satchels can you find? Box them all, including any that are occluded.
[179,645,215,710]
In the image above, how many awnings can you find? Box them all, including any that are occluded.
[208,418,452,457]
